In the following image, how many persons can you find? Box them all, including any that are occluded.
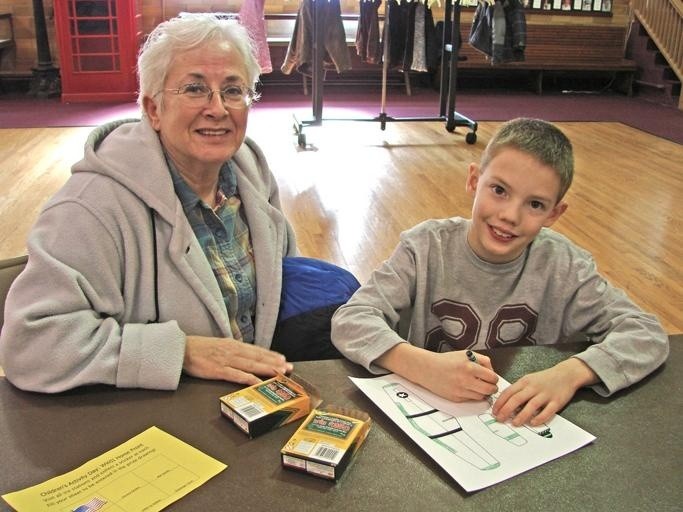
[0,11,304,394]
[330,117,669,427]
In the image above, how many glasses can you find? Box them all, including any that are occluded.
[164,82,248,109]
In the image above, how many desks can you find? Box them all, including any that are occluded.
[0,332,680,511]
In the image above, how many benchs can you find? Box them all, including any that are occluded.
[449,22,637,97]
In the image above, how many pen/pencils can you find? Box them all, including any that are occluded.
[466,350,478,363]
